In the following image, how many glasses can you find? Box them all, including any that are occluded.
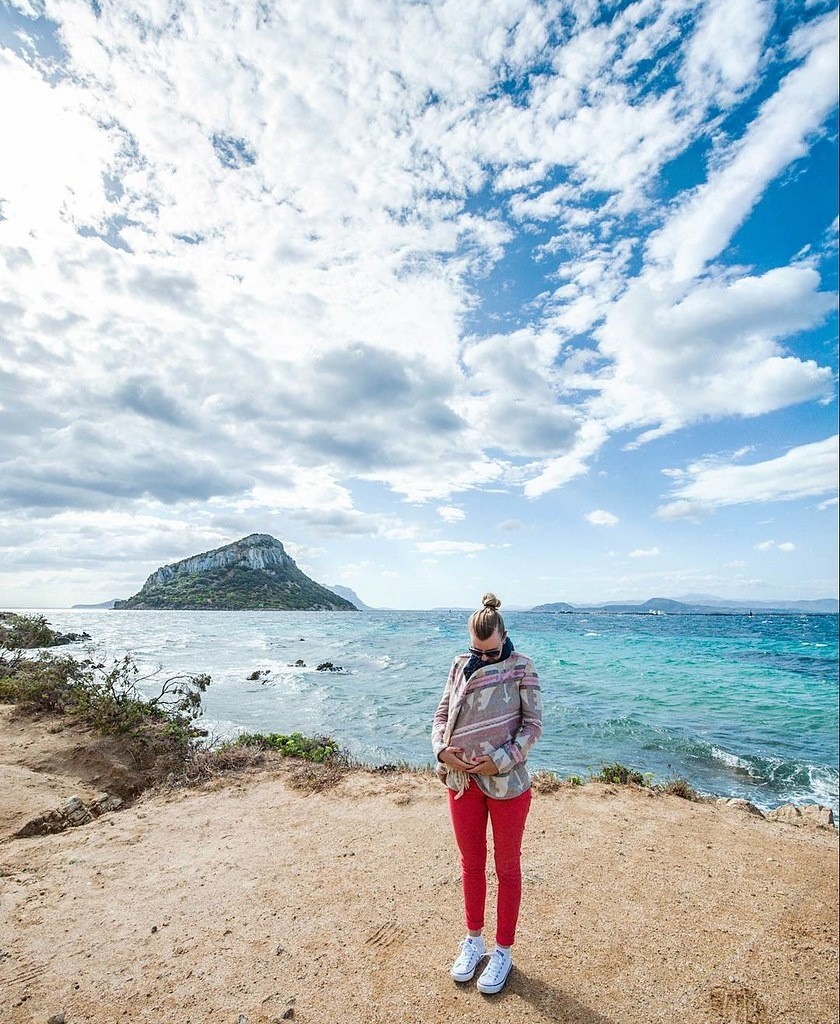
[469,646,501,657]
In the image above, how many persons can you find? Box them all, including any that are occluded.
[432,592,545,995]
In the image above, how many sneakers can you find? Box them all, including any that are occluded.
[451,937,512,994]
